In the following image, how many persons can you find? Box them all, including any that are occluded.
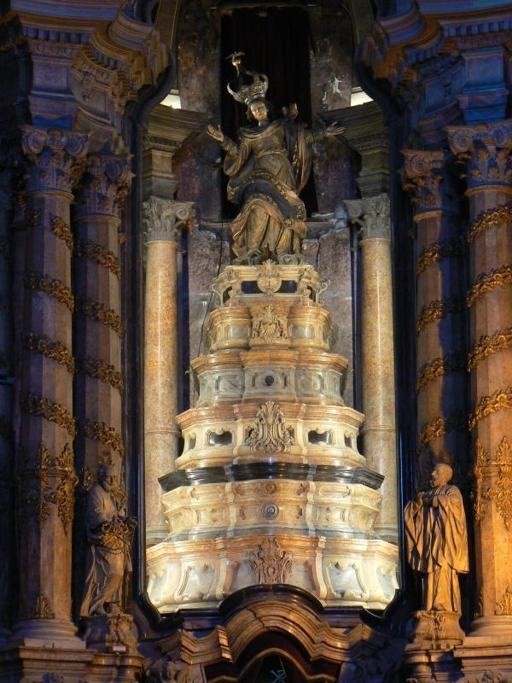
[204,95,347,264]
[76,463,137,617]
[399,461,471,614]
